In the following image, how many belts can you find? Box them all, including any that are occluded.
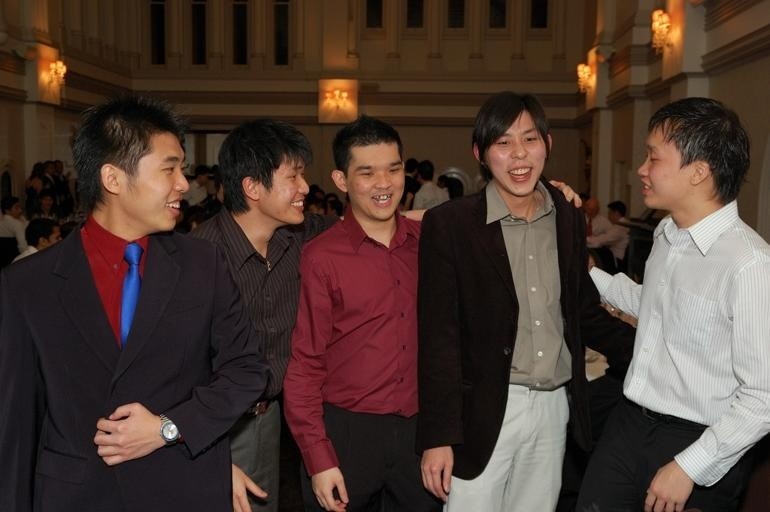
[243,400,268,419]
[626,400,701,425]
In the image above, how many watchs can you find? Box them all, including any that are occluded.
[157,413,179,448]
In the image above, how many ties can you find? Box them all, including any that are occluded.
[120,243,144,349]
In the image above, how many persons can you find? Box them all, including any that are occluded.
[188,113,583,511]
[417,91,637,511]
[579,192,631,276]
[303,184,344,217]
[0,98,267,510]
[283,113,422,511]
[158,163,228,237]
[2,160,86,270]
[398,158,453,221]
[586,95,768,511]
